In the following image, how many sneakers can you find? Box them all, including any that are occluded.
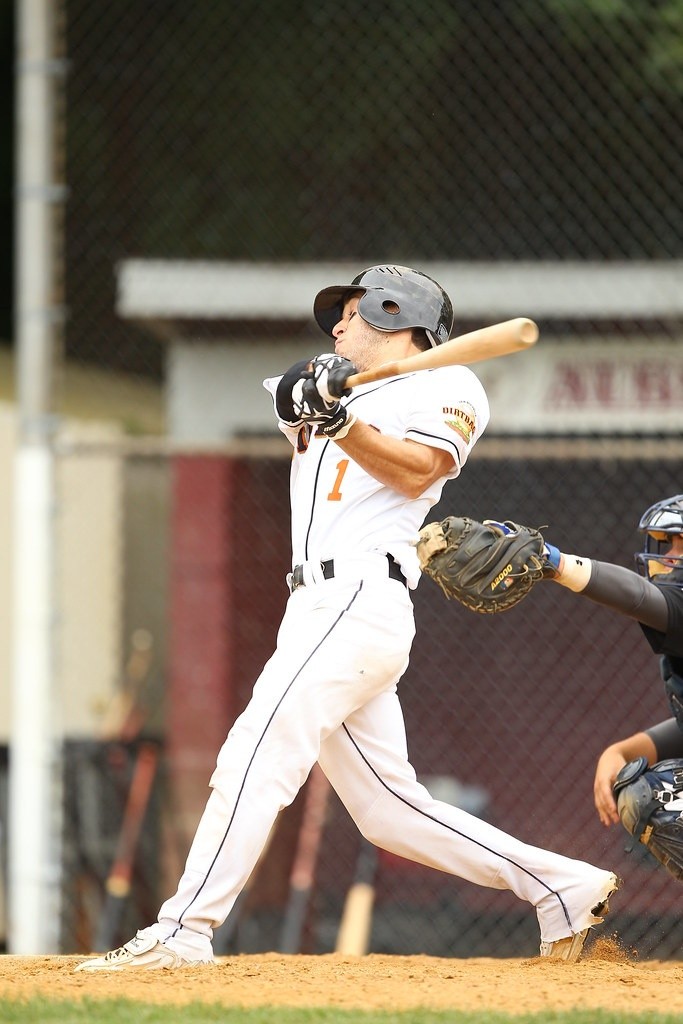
[75,932,214,974]
[541,876,616,962]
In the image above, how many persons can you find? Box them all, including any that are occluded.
[73,265,622,974]
[485,495,683,885]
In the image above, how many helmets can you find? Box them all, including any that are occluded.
[638,494,683,540]
[313,263,454,351]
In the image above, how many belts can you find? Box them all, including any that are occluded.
[289,558,406,590]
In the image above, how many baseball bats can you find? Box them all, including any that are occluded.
[342,317,539,389]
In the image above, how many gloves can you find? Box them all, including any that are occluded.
[263,354,357,430]
[292,370,356,441]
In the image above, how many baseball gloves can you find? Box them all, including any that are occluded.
[415,513,547,614]
